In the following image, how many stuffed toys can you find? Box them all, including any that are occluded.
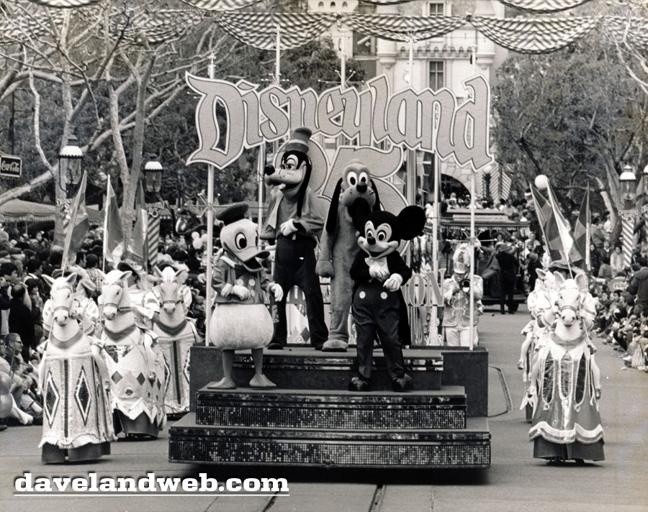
[261,127,329,349]
[209,203,285,390]
[315,157,389,350]
[344,197,426,391]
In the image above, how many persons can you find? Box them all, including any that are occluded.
[0,209,647,437]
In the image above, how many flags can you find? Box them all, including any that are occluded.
[105,176,122,266]
[61,172,91,257]
[570,190,589,265]
[133,182,148,260]
[544,179,574,256]
[530,185,583,265]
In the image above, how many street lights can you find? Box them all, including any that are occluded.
[140,150,163,278]
[619,164,637,270]
[59,134,82,272]
[642,164,647,262]
